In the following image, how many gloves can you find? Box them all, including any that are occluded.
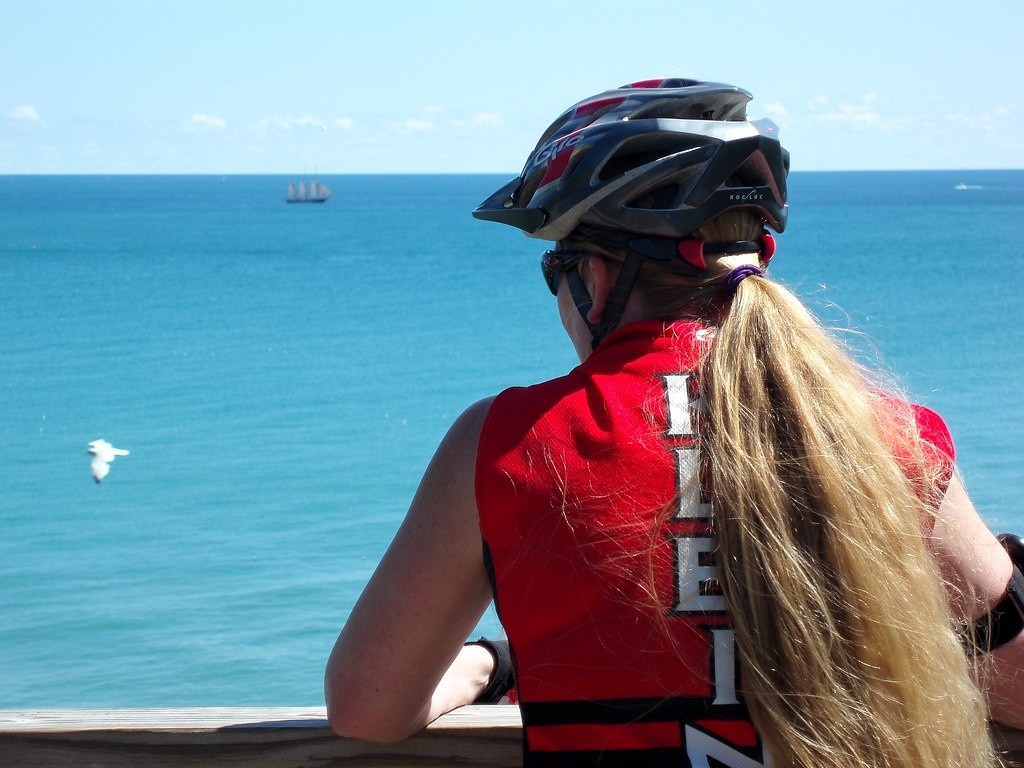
[462,637,521,706]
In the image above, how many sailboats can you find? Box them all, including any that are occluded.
[285,164,333,203]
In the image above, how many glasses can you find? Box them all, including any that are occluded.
[540,247,614,297]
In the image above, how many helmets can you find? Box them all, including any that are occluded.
[471,78,790,250]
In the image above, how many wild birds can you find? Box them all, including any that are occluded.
[86,439,129,483]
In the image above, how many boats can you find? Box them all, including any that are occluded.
[954,183,968,190]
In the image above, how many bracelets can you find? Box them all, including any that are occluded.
[465,639,514,706]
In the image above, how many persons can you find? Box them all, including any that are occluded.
[323,77,1024,768]
